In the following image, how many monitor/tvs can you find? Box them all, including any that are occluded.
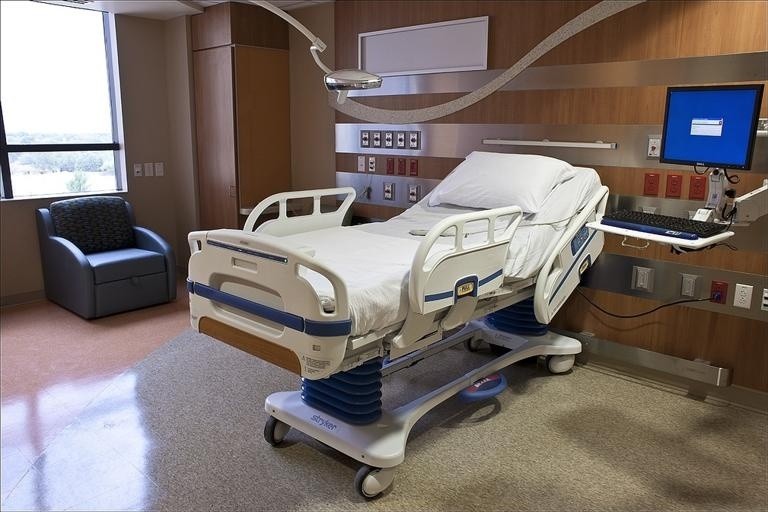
[659,83,765,171]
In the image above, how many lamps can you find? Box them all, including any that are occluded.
[248,0,384,106]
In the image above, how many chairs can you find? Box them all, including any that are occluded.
[36,194,177,319]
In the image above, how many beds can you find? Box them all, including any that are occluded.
[186,149,611,504]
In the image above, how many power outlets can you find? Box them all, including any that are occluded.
[733,283,754,311]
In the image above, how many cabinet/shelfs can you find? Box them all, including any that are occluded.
[192,44,291,231]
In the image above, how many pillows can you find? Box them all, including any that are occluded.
[431,152,574,213]
[49,195,137,255]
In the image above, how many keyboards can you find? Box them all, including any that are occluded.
[602,208,728,239]
